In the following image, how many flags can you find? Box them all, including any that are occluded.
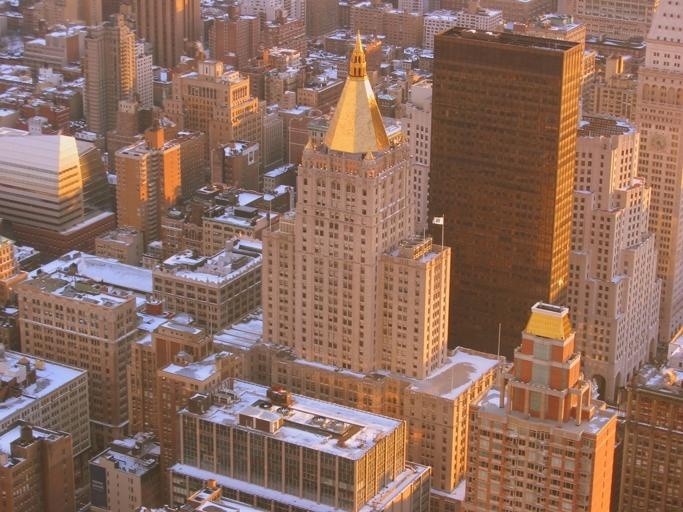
[432,216,445,226]
